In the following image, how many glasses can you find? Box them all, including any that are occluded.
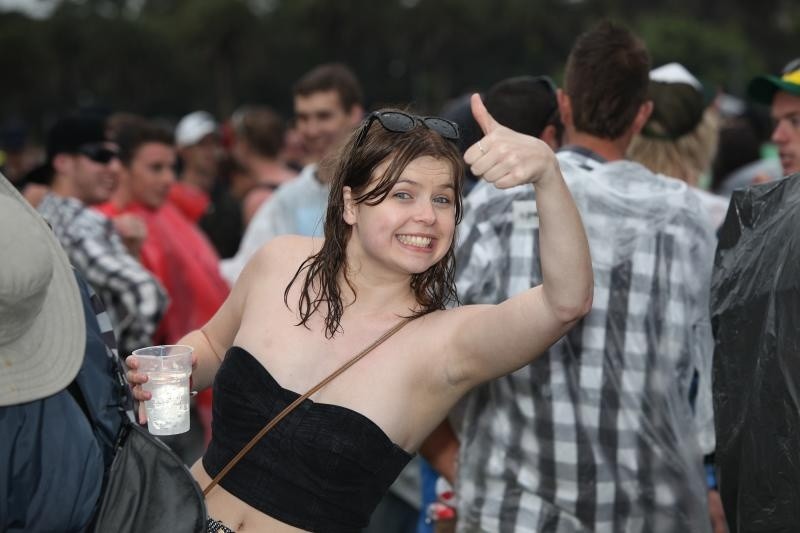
[77,147,131,168]
[354,111,460,153]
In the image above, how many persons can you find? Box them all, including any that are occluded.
[431,23,714,533]
[125,92,593,533]
[0,62,800,533]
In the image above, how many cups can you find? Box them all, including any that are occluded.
[131,344,194,435]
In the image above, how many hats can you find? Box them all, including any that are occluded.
[175,110,218,149]
[0,175,86,405]
[763,58,800,98]
[638,58,718,139]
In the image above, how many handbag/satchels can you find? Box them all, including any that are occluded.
[89,422,207,533]
